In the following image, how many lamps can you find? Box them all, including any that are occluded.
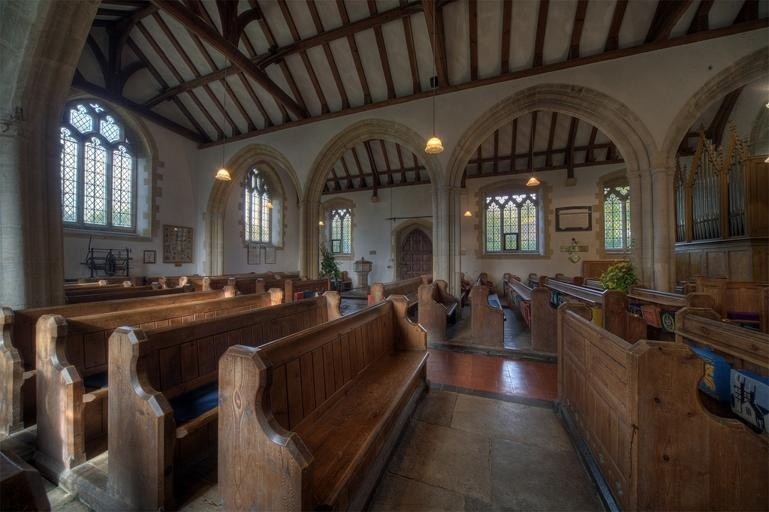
[424,73,445,158]
[217,99,234,184]
[464,165,473,216]
[528,150,541,188]
[266,193,274,209]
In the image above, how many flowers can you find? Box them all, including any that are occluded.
[597,262,638,291]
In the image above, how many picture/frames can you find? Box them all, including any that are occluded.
[143,249,155,264]
[554,205,592,232]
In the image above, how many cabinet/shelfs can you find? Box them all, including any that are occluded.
[581,260,610,276]
[79,247,135,278]
